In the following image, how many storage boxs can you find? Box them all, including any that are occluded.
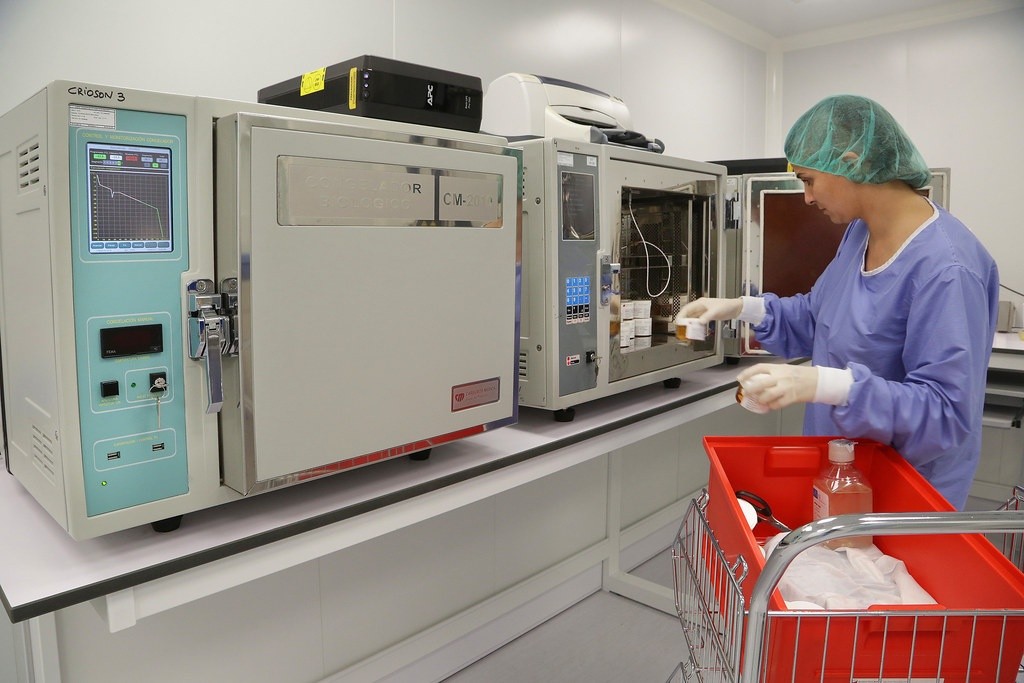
[700,433,1024,683]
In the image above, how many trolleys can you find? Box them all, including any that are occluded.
[664,483,1023,683]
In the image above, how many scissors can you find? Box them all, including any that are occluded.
[734,487,793,532]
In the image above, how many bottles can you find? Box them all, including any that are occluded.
[812,439,872,550]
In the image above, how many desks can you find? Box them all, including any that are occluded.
[0,329,1024,683]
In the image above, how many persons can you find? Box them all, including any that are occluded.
[674,95,1000,514]
